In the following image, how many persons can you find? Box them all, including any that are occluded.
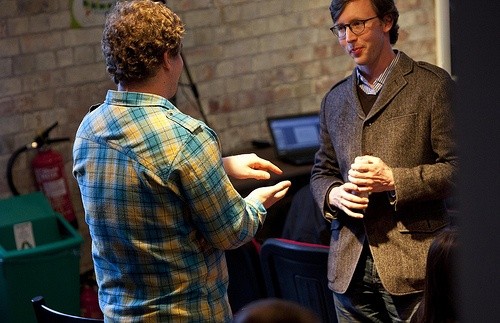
[310,0,473,322]
[279,177,333,258]
[72,0,291,322]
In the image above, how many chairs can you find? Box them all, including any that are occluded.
[221,234,338,323]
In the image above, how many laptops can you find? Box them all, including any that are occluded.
[267,111,321,167]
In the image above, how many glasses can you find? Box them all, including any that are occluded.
[330,15,380,40]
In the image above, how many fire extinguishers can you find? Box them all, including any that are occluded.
[5,119,80,238]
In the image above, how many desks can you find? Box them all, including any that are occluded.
[228,148,314,234]
